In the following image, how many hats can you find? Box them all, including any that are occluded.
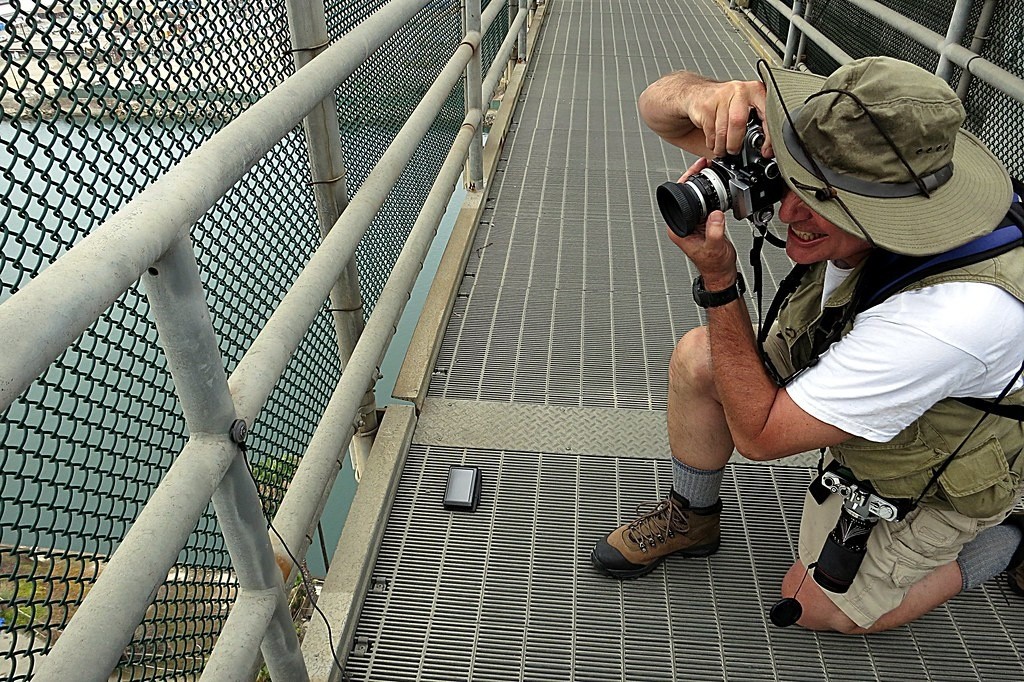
[758,56,1014,255]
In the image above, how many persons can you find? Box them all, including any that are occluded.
[590,57,1024,634]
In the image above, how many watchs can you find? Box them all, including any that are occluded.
[692,272,746,308]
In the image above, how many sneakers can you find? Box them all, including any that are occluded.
[589,488,723,582]
[1004,498,1024,598]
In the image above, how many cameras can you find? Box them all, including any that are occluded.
[657,107,790,238]
[808,467,916,594]
[443,465,477,507]
